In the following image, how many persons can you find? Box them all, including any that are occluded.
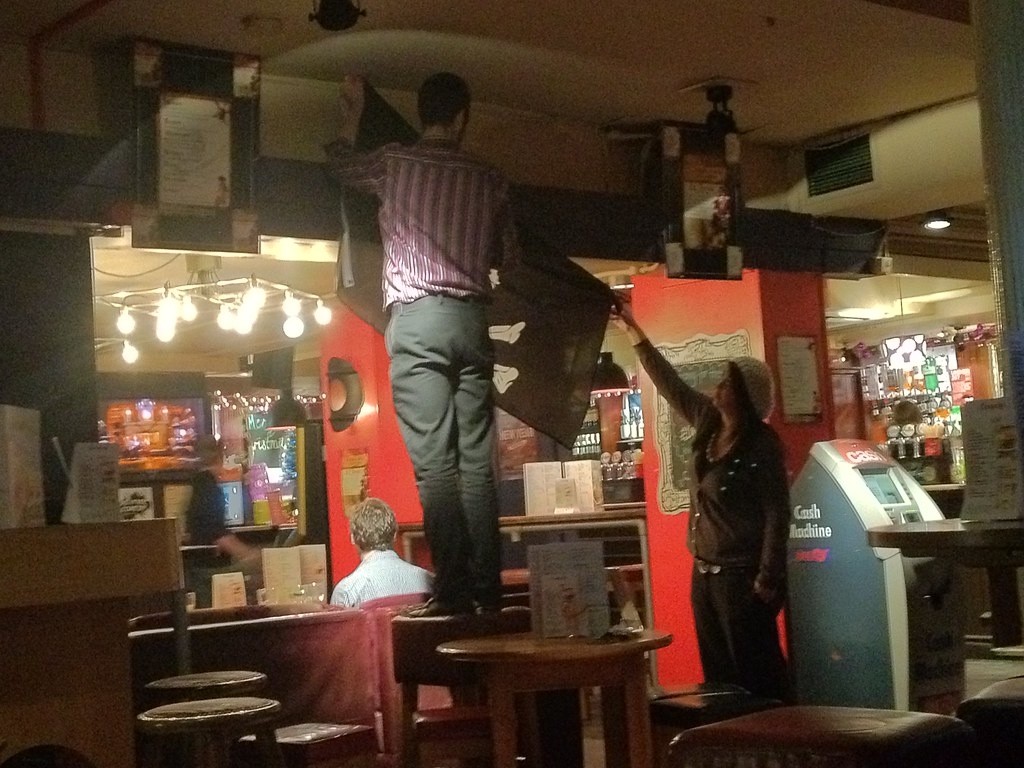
[607,301,794,696]
[182,434,260,609]
[324,73,522,616]
[895,400,922,425]
[329,497,436,610]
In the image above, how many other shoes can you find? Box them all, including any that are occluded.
[409,595,474,615]
[473,599,503,620]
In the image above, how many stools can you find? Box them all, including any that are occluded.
[143,669,271,761]
[668,706,977,768]
[239,722,377,768]
[135,696,281,767]
[958,675,1023,768]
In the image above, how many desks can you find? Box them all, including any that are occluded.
[442,629,677,767]
[868,513,1024,650]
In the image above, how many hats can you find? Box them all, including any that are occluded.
[730,356,774,419]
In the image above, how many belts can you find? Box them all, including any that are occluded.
[695,559,720,574]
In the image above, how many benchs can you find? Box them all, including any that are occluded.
[129,588,430,767]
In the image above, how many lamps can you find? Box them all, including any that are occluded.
[920,208,954,232]
[591,327,631,393]
[96,253,332,362]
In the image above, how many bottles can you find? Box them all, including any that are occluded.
[948,446,966,484]
[572,396,601,455]
[620,406,644,438]
[861,363,904,400]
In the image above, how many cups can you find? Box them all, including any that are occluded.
[925,437,943,456]
[253,500,271,525]
[184,592,196,610]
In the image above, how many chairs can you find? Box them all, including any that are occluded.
[390,607,534,767]
